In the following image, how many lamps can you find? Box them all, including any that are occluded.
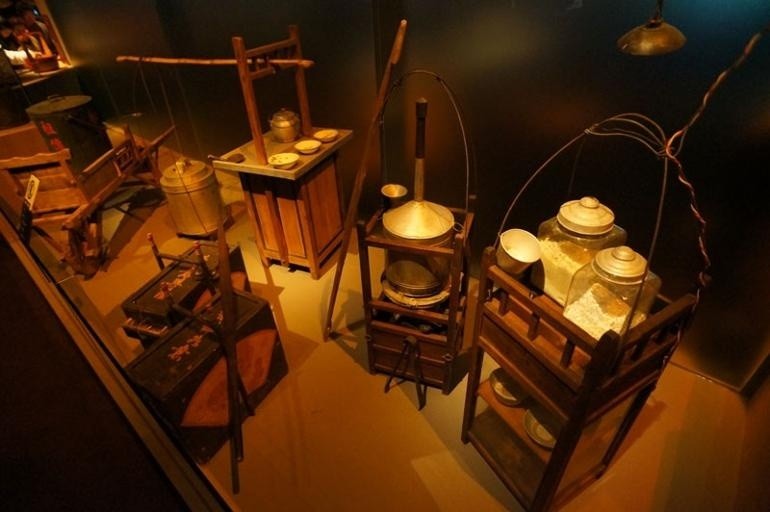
[613,1,685,57]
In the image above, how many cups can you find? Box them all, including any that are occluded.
[494,229,543,275]
[380,184,408,211]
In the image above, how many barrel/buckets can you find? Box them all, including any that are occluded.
[159,158,230,238]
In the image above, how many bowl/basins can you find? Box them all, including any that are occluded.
[522,406,557,450]
[489,367,531,406]
[267,128,338,170]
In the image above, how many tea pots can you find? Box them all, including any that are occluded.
[268,107,302,143]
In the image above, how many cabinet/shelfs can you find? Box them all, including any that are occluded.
[461,239,693,509]
[350,189,470,395]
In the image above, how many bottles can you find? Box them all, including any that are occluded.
[532,195,663,342]
